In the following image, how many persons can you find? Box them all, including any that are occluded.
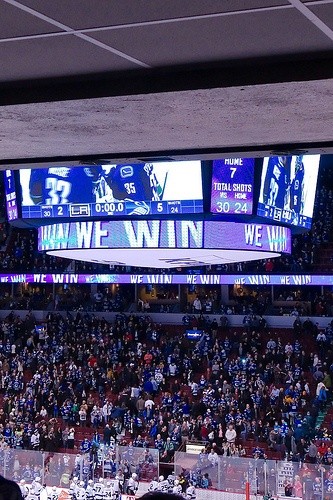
[28,165,107,205]
[0,187,333,500]
[108,163,164,202]
[262,155,305,214]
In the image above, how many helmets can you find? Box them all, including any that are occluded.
[18,466,187,497]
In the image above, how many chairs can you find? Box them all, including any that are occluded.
[0,323,333,465]
